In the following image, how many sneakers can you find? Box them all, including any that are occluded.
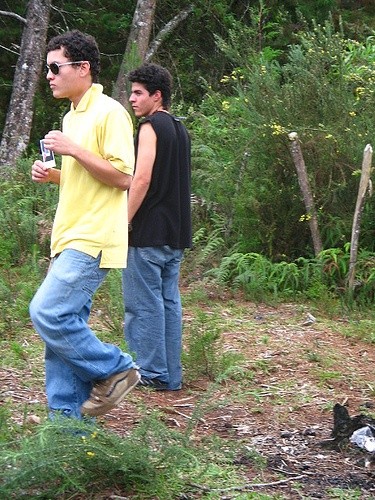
[134,377,168,390]
[80,367,141,416]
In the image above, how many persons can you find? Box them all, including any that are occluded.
[123,61,193,391]
[28,30,142,440]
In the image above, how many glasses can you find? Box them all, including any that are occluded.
[45,61,82,75]
